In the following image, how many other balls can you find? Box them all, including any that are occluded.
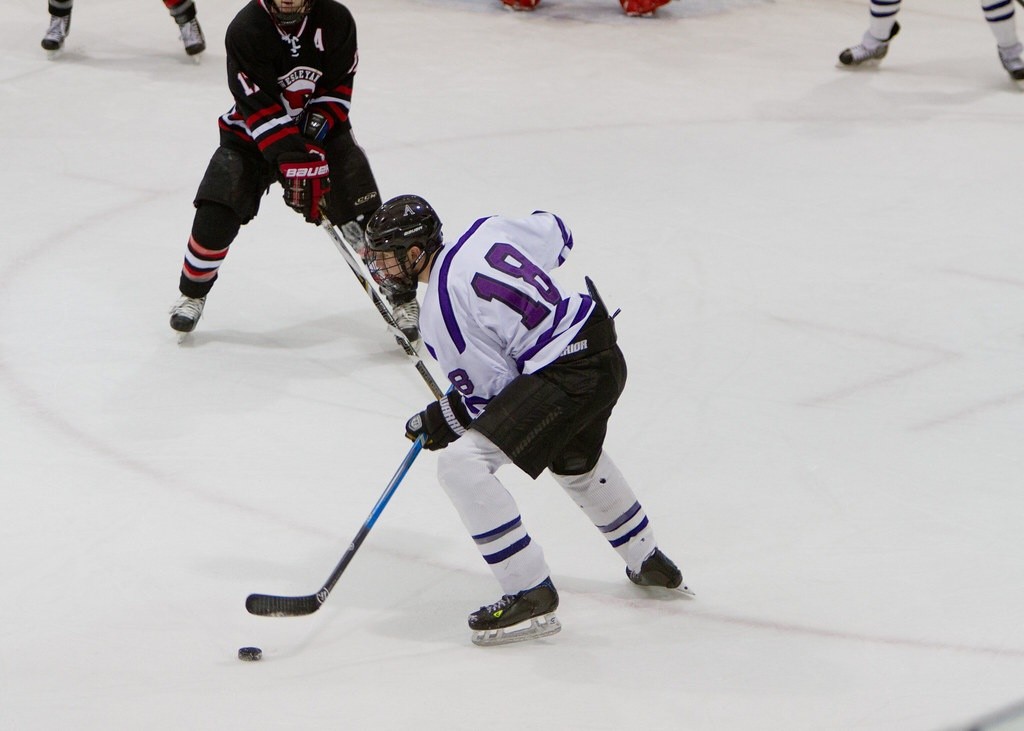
[237,647,264,663]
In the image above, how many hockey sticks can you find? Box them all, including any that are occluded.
[245,383,464,618]
[314,204,449,402]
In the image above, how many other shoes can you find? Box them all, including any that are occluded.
[501,0,540,11]
[619,0,671,16]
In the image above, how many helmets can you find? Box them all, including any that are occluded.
[266,0,312,33]
[364,194,443,303]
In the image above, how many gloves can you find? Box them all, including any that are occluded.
[405,390,474,451]
[274,141,331,227]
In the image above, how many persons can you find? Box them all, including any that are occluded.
[169,1,422,350]
[39,1,207,58]
[363,192,684,632]
[836,0,1024,81]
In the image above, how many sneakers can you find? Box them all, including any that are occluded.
[835,20,900,71]
[468,576,562,649]
[997,42,1024,90]
[41,14,71,60]
[378,286,423,356]
[178,16,206,63]
[626,546,696,599]
[168,291,206,346]
